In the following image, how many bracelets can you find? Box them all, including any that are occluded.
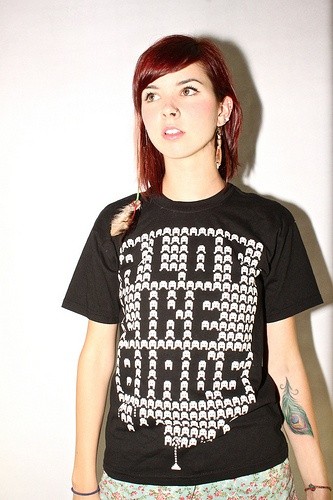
[71,487,100,496]
[304,486,332,492]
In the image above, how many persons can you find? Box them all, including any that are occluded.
[59,34,333,500]
